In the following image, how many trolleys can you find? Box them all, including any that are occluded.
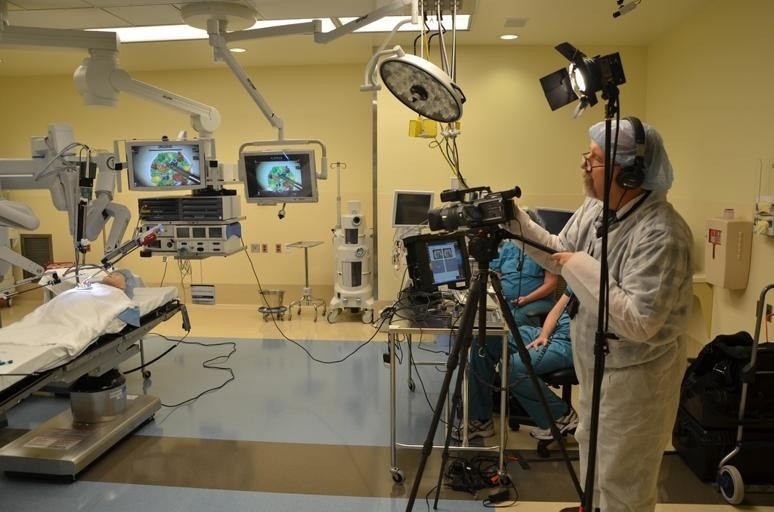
[387,304,515,488]
[672,284,774,505]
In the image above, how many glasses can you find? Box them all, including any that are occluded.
[580,151,619,173]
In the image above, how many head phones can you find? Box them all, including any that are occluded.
[613,115,649,190]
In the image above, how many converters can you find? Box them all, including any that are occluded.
[484,485,511,502]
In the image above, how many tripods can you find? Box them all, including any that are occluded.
[404,265,589,512]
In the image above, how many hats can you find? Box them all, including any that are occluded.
[113,268,135,300]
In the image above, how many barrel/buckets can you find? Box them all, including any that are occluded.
[258,289,285,310]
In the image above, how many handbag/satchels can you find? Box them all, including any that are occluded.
[672,333,754,485]
[679,331,773,427]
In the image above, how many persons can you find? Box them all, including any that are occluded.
[447,278,580,445]
[497,113,695,512]
[464,204,558,328]
[102,267,137,300]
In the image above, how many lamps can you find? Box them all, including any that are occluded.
[359,19,466,124]
[539,41,626,119]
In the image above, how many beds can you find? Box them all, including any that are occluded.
[0,285,191,484]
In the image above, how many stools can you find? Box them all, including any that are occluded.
[511,366,580,457]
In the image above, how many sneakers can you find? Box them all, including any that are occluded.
[529,405,579,440]
[451,417,494,441]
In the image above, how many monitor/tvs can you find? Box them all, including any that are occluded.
[425,233,472,289]
[125,140,206,191]
[393,191,434,229]
[240,148,319,204]
